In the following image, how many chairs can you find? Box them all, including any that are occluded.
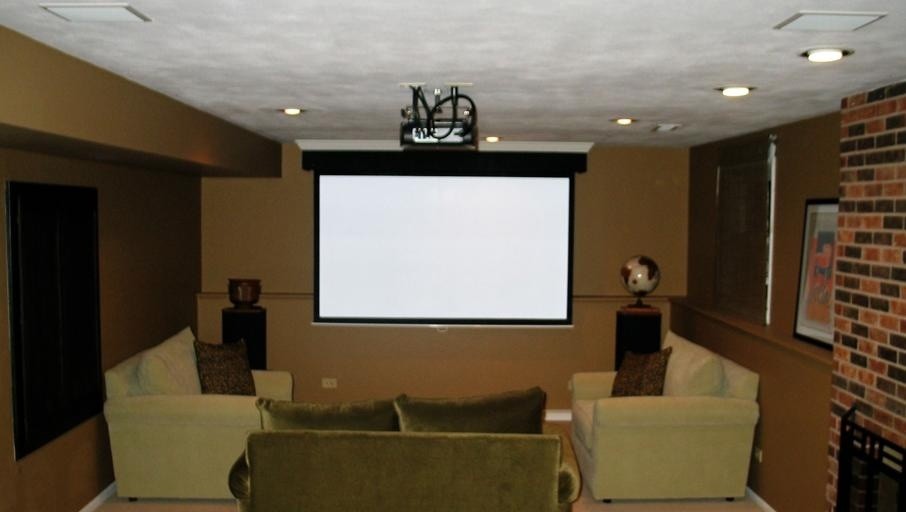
[227,426,582,512]
[568,329,761,504]
[103,325,294,501]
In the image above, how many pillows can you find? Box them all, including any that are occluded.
[193,337,256,397]
[610,345,672,398]
[255,385,549,435]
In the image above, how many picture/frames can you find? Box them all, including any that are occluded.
[792,196,840,352]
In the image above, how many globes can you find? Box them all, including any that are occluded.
[620,255,660,308]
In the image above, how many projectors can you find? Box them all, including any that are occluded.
[399,118,477,147]
